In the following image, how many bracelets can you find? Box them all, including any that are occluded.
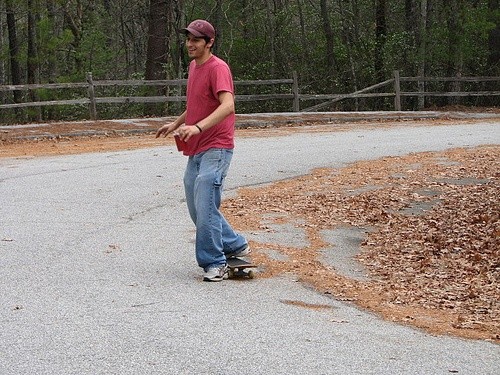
[195,124,203,132]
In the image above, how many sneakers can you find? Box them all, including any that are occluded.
[226,244,251,259]
[204,263,229,282]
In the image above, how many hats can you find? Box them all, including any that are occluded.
[179,19,215,39]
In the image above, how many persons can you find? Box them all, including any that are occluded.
[155,20,252,282]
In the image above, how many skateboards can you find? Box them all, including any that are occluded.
[225,257,257,278]
